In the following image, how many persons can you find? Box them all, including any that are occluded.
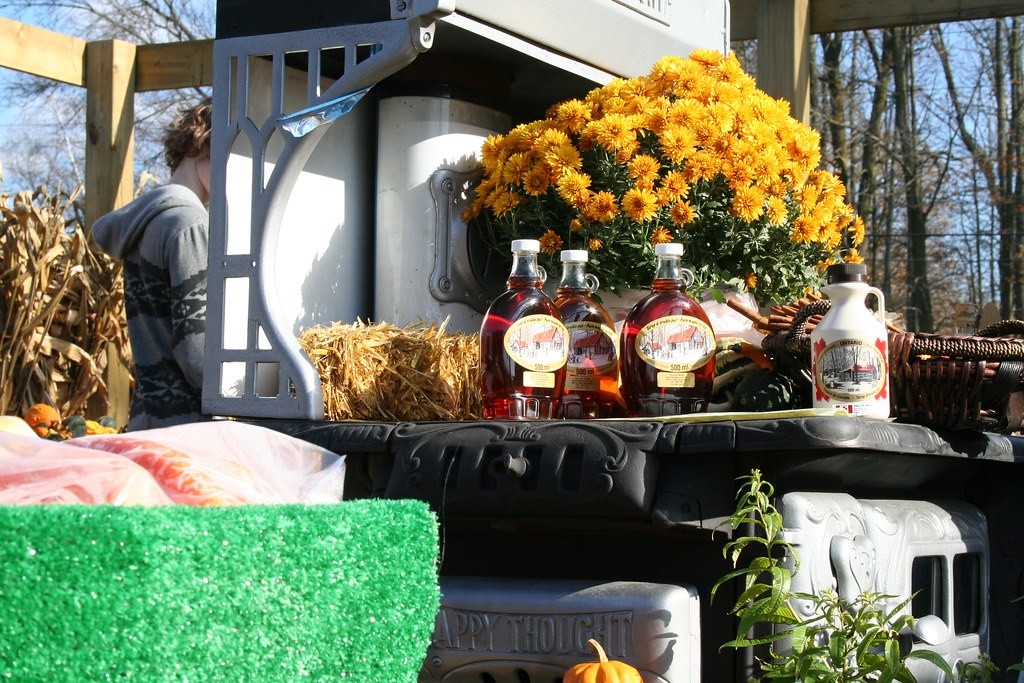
[91,93,212,430]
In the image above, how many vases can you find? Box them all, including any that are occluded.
[505,272,766,368]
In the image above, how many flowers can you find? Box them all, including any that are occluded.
[464,52,867,300]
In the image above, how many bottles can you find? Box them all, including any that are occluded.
[479,239,569,420]
[811,264,891,420]
[553,250,620,418]
[620,244,715,417]
